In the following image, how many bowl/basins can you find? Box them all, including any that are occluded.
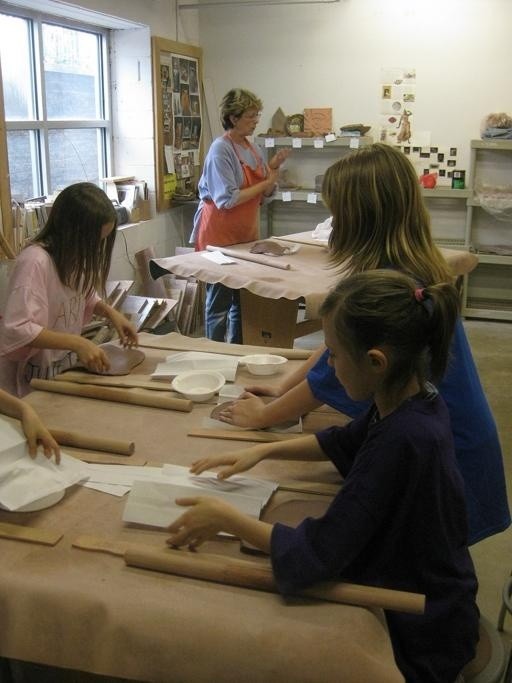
[239,353,287,381]
[173,368,223,404]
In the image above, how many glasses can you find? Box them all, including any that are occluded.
[235,110,262,122]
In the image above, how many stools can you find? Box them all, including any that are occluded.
[458,612,512,682]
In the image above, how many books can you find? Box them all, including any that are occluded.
[11,199,49,252]
[118,180,149,204]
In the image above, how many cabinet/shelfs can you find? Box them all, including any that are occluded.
[459,139,512,322]
[253,134,374,239]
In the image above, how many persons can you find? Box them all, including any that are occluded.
[0,182,139,397]
[165,270,481,683]
[1,389,64,466]
[187,87,293,347]
[219,141,512,550]
[395,109,412,145]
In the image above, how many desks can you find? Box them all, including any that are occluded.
[1,331,409,683]
[148,221,480,351]
